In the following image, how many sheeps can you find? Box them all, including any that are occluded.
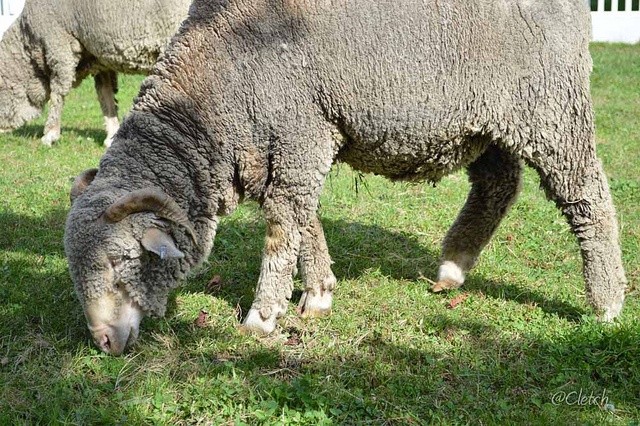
[63,2,629,355]
[1,0,193,148]
